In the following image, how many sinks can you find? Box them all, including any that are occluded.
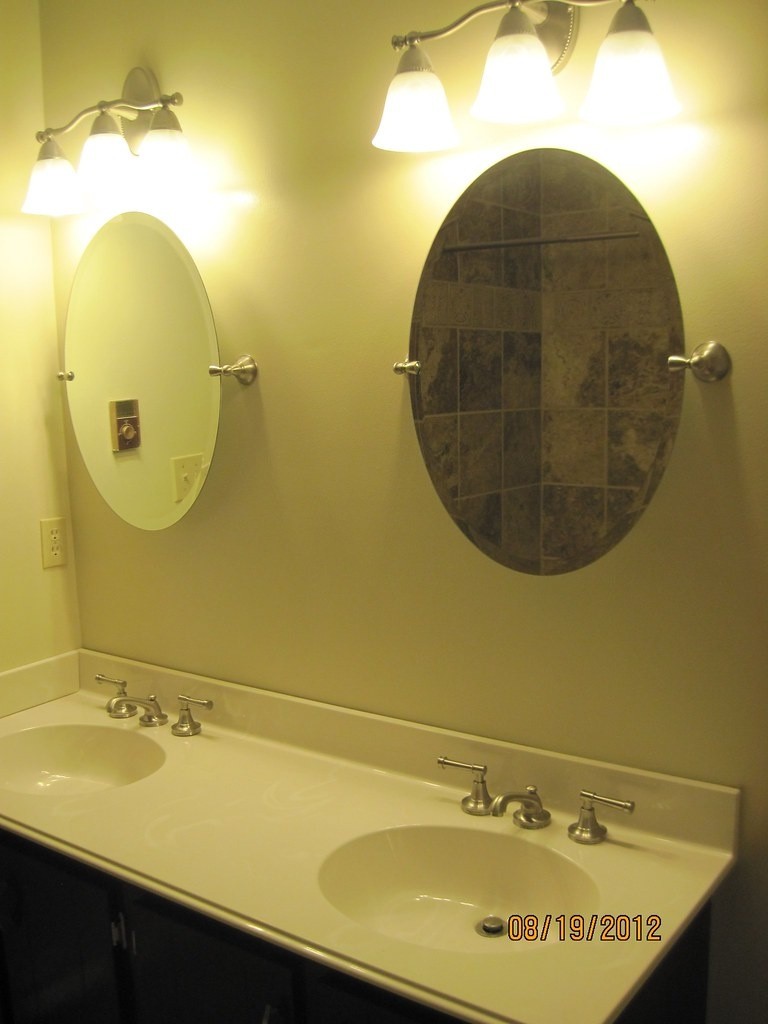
[0,724,166,795]
[318,825,601,956]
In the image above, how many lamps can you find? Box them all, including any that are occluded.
[372,0,673,152]
[22,68,196,219]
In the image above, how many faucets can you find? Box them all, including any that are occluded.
[489,785,552,829]
[106,692,168,728]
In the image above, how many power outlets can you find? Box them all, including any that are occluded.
[40,517,66,568]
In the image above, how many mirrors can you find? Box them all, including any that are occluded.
[406,147,682,575]
[62,212,219,532]
